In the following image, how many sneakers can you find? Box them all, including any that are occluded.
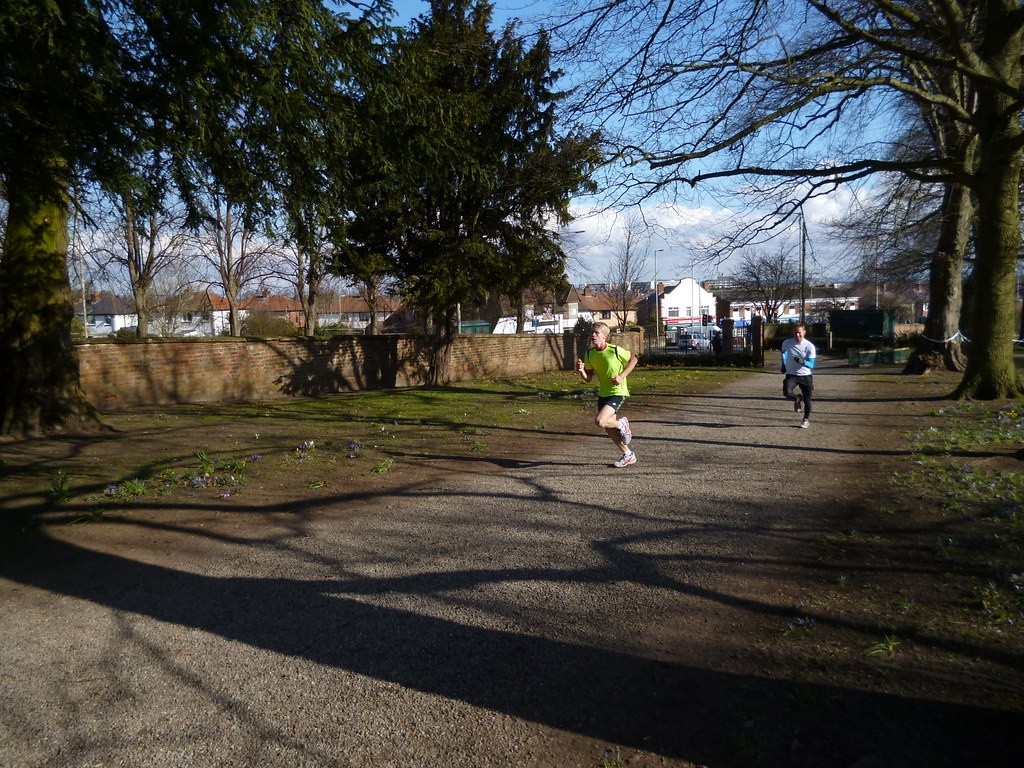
[618,416,632,445]
[613,452,637,468]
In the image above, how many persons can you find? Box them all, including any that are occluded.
[781,323,816,429]
[712,333,721,355]
[576,322,638,468]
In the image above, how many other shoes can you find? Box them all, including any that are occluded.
[800,417,809,429]
[794,394,803,413]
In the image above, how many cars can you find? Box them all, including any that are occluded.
[678,333,711,349]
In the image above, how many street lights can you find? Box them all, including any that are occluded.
[654,249,664,342]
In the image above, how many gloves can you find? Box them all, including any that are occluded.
[780,367,787,374]
[794,356,804,365]
